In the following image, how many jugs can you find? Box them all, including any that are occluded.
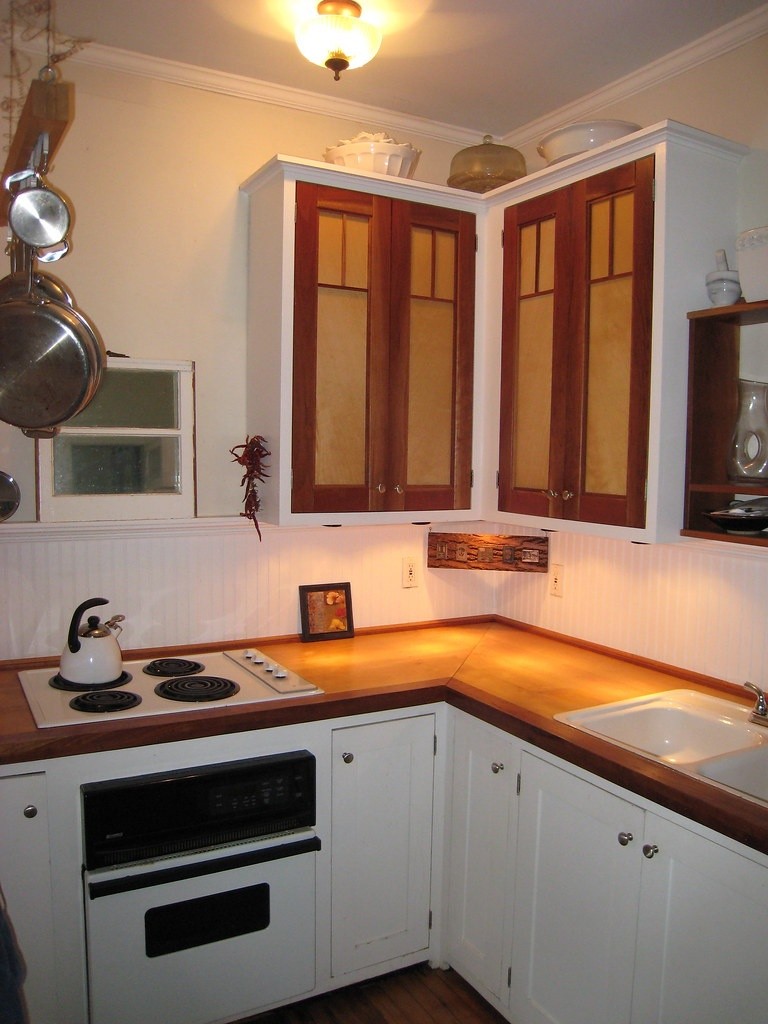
[60,598,125,684]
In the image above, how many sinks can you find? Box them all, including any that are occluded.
[556,688,768,764]
[698,743,768,801]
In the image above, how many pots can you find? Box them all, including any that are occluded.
[0,169,106,439]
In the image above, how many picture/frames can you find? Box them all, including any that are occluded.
[299,582,355,642]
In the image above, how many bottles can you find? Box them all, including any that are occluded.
[725,377,768,486]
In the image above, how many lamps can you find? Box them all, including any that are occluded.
[295,0,385,80]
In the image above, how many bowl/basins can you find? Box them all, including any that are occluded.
[735,226,768,303]
[701,511,768,534]
[537,119,642,165]
[325,142,422,179]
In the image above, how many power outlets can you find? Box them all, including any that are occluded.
[403,555,418,588]
[549,562,564,598]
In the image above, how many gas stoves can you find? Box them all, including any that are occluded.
[17,648,324,728]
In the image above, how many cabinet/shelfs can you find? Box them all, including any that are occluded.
[443,704,767,1024]
[483,118,755,546]
[0,759,92,1024]
[238,153,487,527]
[679,299,768,549]
[330,702,442,987]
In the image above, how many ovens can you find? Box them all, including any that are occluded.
[80,749,322,1024]
